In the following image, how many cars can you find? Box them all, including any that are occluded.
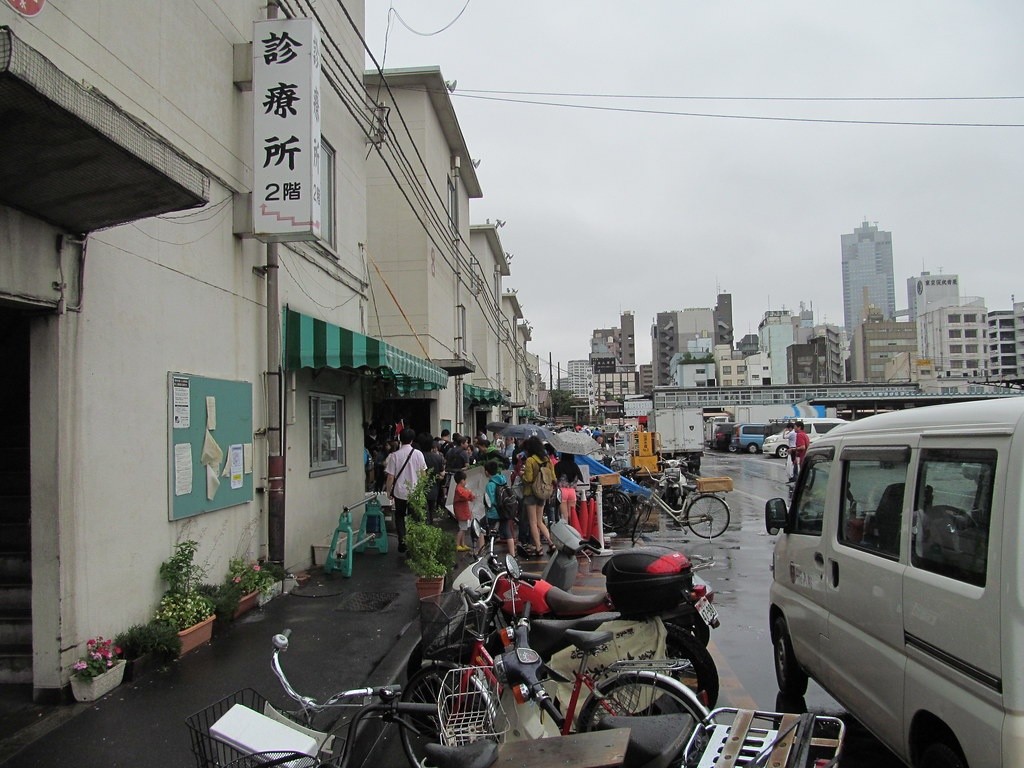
[703,414,852,459]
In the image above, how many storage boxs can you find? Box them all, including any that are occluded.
[603,546,693,608]
[599,473,621,485]
[697,475,734,492]
[210,703,318,768]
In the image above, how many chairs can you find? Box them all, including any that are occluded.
[871,483,905,552]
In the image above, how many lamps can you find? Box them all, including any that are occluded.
[525,319,531,325]
[505,251,514,259]
[473,158,481,169]
[496,219,506,228]
[512,288,518,293]
[444,80,457,93]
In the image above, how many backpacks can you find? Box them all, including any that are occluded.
[529,456,554,499]
[488,450,510,470]
[489,479,518,520]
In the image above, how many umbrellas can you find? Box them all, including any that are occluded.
[548,431,602,456]
[500,424,550,440]
[486,422,507,431]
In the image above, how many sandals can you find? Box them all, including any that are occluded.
[546,544,556,554]
[530,548,543,558]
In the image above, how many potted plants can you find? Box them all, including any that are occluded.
[205,586,240,638]
[144,625,183,669]
[403,467,447,599]
[119,626,148,679]
[440,532,456,586]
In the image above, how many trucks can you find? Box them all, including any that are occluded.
[644,406,705,471]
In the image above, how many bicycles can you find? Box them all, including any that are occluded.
[630,465,731,543]
[182,443,849,768]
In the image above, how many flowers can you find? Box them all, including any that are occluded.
[148,540,214,627]
[74,635,122,680]
[231,558,273,593]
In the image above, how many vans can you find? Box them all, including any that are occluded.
[762,394,1024,768]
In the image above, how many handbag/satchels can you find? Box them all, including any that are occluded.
[389,478,396,498]
[556,487,562,504]
[785,454,794,478]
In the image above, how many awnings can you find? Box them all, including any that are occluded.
[518,407,536,418]
[463,383,503,409]
[282,303,448,396]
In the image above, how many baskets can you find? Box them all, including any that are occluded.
[419,589,494,658]
[665,471,680,484]
[437,664,510,747]
[219,750,319,767]
[183,686,346,768]
[637,480,664,506]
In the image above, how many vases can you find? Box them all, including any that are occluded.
[257,578,283,608]
[66,662,126,701]
[236,589,266,615]
[178,615,216,655]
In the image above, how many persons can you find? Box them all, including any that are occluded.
[533,420,605,444]
[383,428,428,557]
[519,436,557,556]
[554,454,584,525]
[363,418,519,490]
[453,471,475,552]
[468,460,516,557]
[783,421,810,481]
[417,435,445,525]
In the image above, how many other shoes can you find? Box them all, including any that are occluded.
[462,545,472,550]
[513,555,518,562]
[455,545,466,551]
